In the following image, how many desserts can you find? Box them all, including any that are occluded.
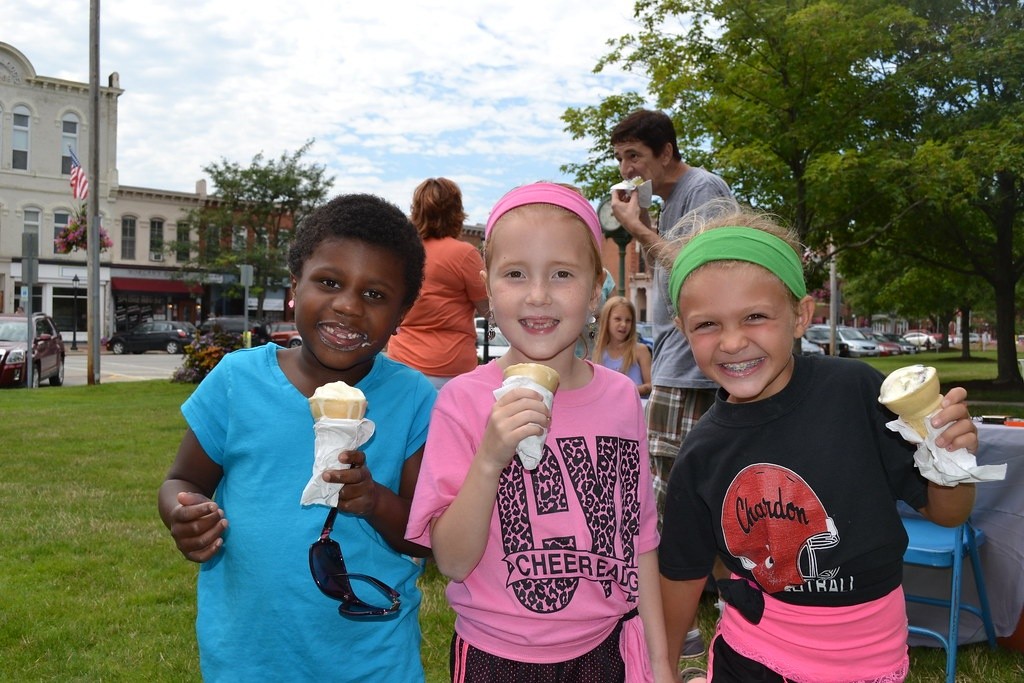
[622,176,644,186]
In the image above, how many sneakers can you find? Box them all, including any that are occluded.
[675,628,706,659]
[680,667,707,683]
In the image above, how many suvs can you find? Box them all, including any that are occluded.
[201,316,302,347]
[1,311,65,389]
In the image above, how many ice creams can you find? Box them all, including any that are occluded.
[878,364,943,439]
[308,379,366,421]
[502,362,560,396]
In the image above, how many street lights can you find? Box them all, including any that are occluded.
[70,273,81,350]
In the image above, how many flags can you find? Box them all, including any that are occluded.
[69,148,89,200]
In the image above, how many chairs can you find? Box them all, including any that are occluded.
[902,514,998,683]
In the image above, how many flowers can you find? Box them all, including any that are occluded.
[53,201,114,255]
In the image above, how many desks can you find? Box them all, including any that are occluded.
[893,416,1024,653]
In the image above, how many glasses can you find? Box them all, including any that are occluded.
[309,506,401,616]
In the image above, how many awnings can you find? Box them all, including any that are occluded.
[111,277,204,293]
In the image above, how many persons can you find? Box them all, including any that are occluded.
[16,307,24,314]
[158,192,439,683]
[404,181,673,683]
[385,177,491,565]
[591,110,741,683]
[657,204,978,683]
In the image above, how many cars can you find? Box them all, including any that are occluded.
[634,323,653,353]
[800,325,981,357]
[475,317,510,346]
[105,321,197,355]
